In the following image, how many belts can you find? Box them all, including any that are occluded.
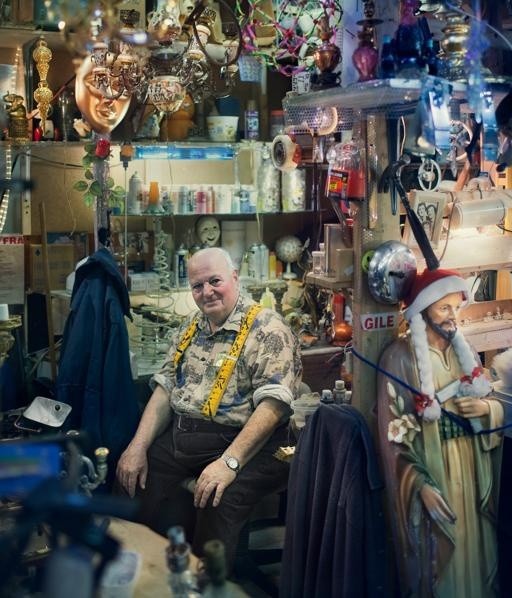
[175,415,237,433]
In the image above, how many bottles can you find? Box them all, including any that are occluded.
[174,242,190,291]
[239,241,278,282]
[321,380,347,407]
[164,527,193,598]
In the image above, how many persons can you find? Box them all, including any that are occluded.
[417,202,427,222]
[421,220,432,239]
[111,246,295,580]
[426,205,436,223]
[377,268,505,598]
[74,53,132,132]
[196,217,221,245]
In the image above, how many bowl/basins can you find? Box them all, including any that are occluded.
[205,115,239,143]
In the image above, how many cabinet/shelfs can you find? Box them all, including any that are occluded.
[106,141,345,392]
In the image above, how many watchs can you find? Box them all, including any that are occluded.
[221,453,241,474]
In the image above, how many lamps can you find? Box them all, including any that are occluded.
[84,1,243,115]
